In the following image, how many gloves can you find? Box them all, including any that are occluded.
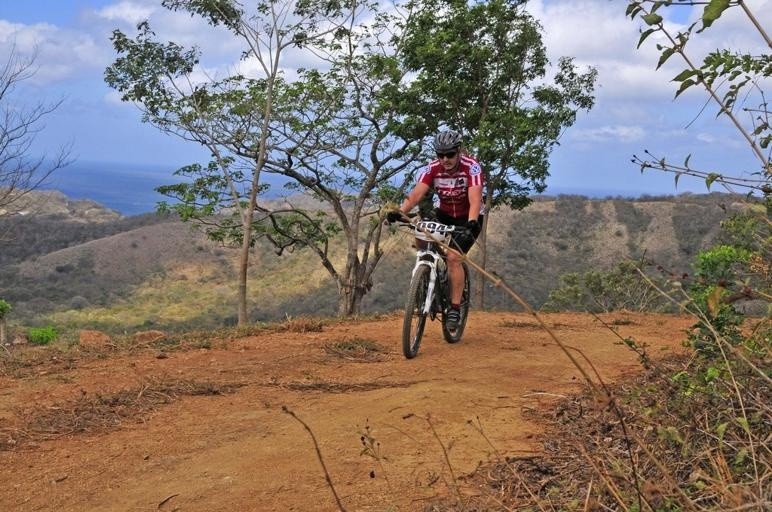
[463,220,481,234]
[387,211,401,223]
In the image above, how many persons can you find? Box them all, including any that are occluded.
[387,130,485,331]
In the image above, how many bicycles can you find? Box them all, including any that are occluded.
[386,208,472,358]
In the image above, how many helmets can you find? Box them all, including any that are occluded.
[432,130,463,150]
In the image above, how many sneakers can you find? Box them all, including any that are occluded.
[446,309,460,329]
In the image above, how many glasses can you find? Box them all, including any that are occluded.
[435,152,457,158]
